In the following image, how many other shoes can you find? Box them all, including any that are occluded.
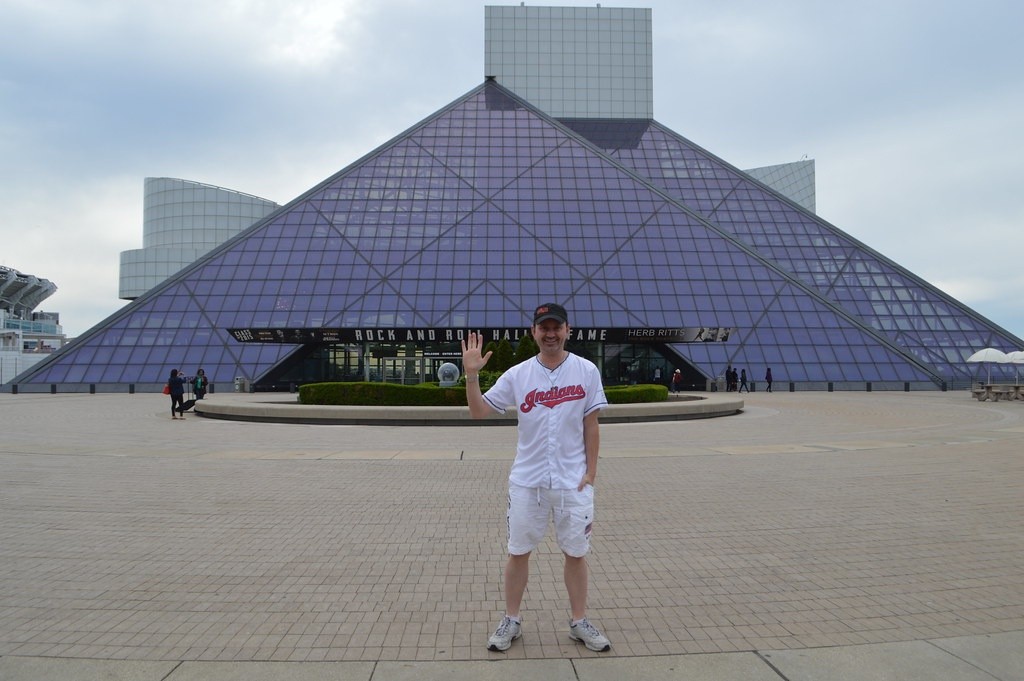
[172,416,178,419]
[180,416,185,419]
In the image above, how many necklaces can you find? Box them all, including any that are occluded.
[537,356,562,393]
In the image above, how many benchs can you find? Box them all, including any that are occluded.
[972,390,1024,400]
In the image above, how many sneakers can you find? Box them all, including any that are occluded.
[568,615,610,652]
[486,615,522,652]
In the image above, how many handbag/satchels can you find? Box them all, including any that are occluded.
[163,384,170,395]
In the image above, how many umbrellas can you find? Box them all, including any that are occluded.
[997,350,1024,385]
[966,347,1013,385]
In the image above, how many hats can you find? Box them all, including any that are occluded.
[534,303,568,323]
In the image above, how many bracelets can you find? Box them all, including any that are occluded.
[464,375,480,381]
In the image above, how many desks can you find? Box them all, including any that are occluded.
[977,385,1000,402]
[1006,386,1024,401]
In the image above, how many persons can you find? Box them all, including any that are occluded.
[168,368,187,419]
[673,369,682,393]
[764,367,772,392]
[653,365,662,384]
[726,365,738,393]
[462,303,614,653]
[738,369,749,393]
[190,369,208,399]
